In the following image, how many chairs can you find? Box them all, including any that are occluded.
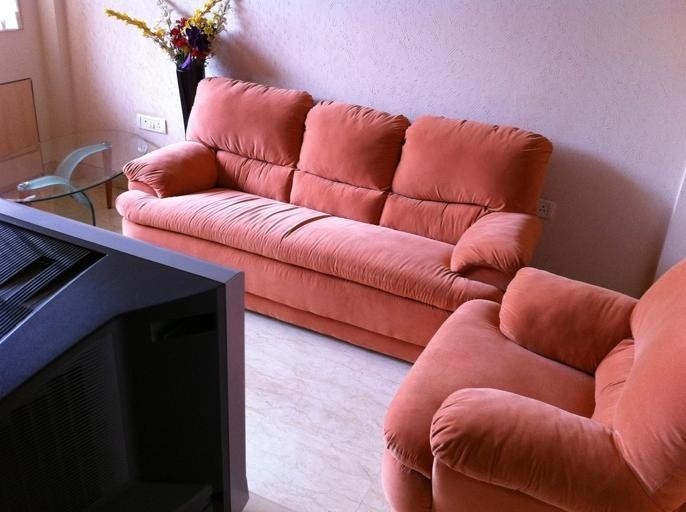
[384,257,686,512]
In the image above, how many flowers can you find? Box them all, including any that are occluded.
[105,0,231,72]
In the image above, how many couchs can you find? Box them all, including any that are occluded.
[112,76,554,366]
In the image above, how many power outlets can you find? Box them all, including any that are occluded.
[134,113,167,136]
[537,198,556,219]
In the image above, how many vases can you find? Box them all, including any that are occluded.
[176,68,205,134]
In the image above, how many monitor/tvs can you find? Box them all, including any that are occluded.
[0,197,250,512]
